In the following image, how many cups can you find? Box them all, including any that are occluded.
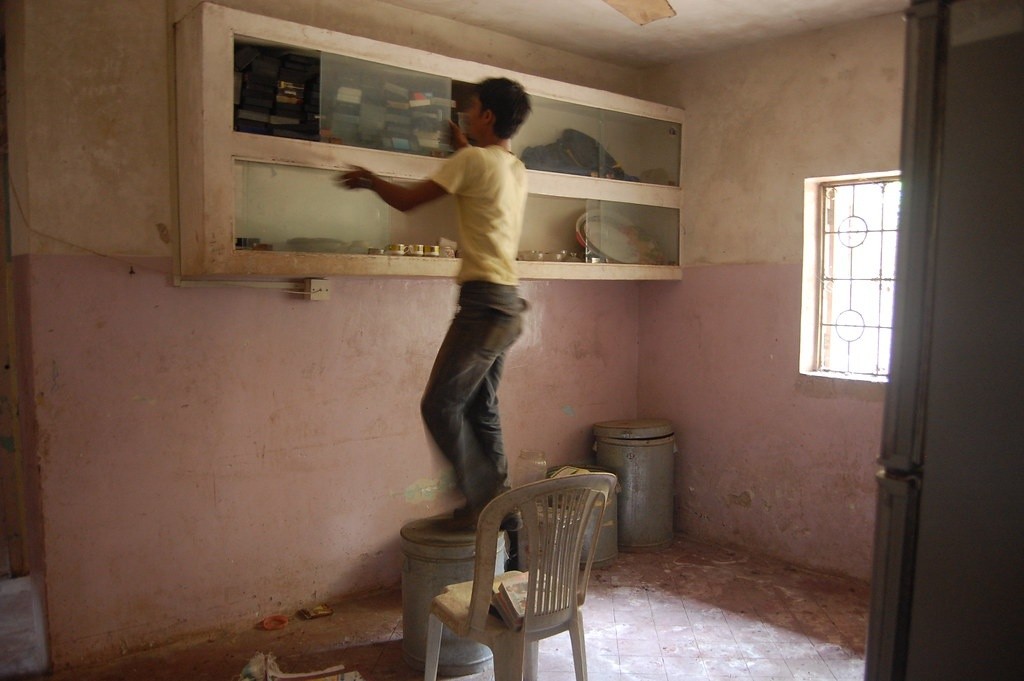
[440,246,464,258]
[368,247,384,255]
[425,245,440,257]
[384,242,410,255]
[409,244,424,256]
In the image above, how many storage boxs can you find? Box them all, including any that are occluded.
[234,42,475,160]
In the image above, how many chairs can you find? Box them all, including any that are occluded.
[425,473,618,681]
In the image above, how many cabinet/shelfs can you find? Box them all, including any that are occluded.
[174,2,685,281]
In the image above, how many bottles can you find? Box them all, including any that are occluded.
[512,448,548,487]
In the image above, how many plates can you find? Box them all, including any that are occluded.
[518,248,567,261]
[583,221,640,263]
[285,236,348,253]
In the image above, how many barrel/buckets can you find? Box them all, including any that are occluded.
[546,464,622,571]
[399,519,510,677]
[0,569,48,678]
[591,418,679,550]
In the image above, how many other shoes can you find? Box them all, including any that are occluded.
[452,501,526,532]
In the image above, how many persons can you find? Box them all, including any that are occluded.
[339,76,532,533]
